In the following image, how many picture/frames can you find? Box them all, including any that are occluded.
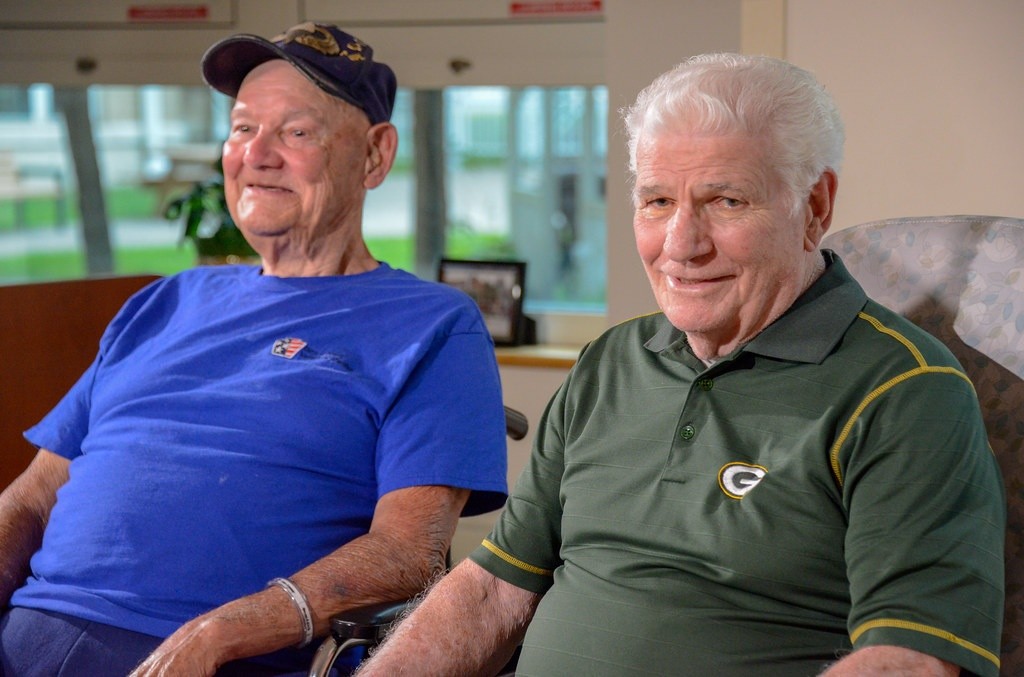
[433,255,529,350]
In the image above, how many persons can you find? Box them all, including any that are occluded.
[350,50,1008,677]
[0,25,511,677]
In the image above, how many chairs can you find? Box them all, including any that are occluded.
[309,405,529,677]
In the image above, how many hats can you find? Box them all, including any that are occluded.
[197,24,399,125]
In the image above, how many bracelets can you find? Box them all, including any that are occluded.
[266,577,314,651]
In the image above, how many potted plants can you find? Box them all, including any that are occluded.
[159,139,262,267]
